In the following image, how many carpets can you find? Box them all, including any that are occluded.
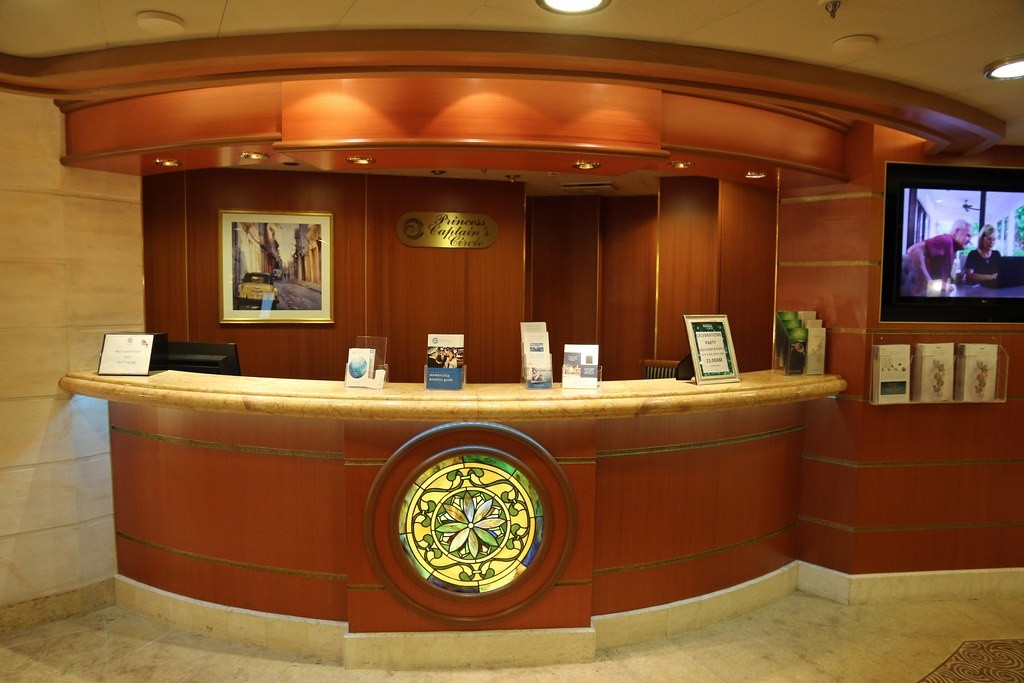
[916,638,1024,683]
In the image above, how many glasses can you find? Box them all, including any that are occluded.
[439,350,444,352]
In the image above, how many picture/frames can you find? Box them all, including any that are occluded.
[683,314,741,386]
[216,208,336,324]
[96,332,155,376]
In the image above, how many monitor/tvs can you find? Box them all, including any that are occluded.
[877,161,1024,324]
[168,341,242,376]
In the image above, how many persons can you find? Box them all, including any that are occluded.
[444,348,458,368]
[964,224,1002,285]
[908,218,973,296]
[437,347,447,364]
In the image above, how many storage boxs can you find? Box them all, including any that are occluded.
[423,364,467,392]
[344,364,389,390]
[561,364,603,392]
[773,311,826,377]
[868,342,1009,404]
[521,354,554,391]
